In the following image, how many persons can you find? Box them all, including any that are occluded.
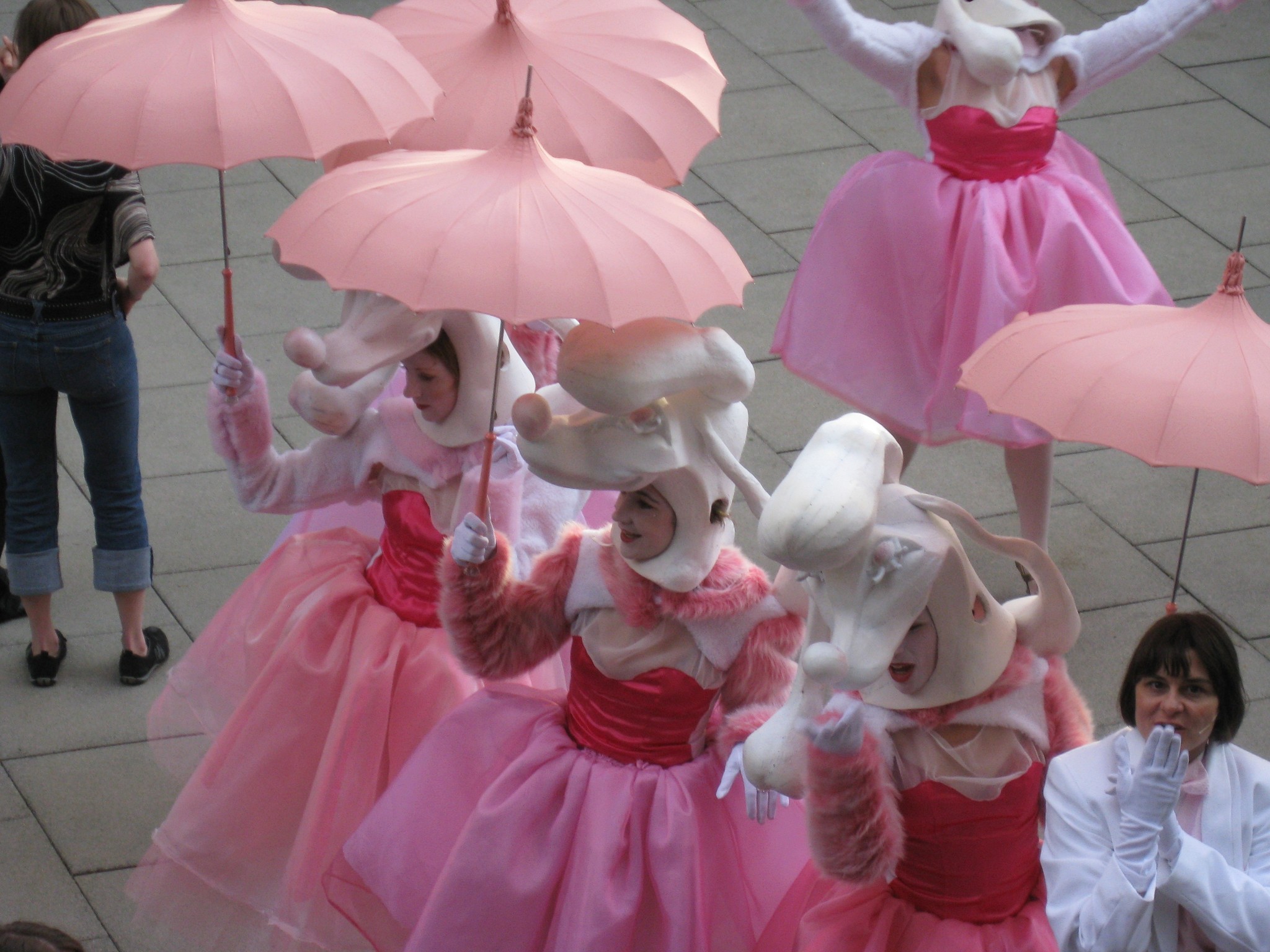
[325,320,809,952]
[1039,613,1270,951]
[770,0,1239,596]
[262,309,594,563]
[126,299,572,950]
[744,413,1090,952]
[0,0,171,686]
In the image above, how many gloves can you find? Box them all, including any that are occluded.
[451,494,498,571]
[795,692,865,755]
[1107,769,1182,865]
[209,325,254,401]
[716,741,788,824]
[1113,725,1188,893]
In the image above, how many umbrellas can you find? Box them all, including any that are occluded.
[0,0,442,397]
[958,217,1270,614]
[266,64,755,577]
[322,0,727,190]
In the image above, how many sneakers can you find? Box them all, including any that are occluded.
[26,629,66,686]
[118,626,170,686]
